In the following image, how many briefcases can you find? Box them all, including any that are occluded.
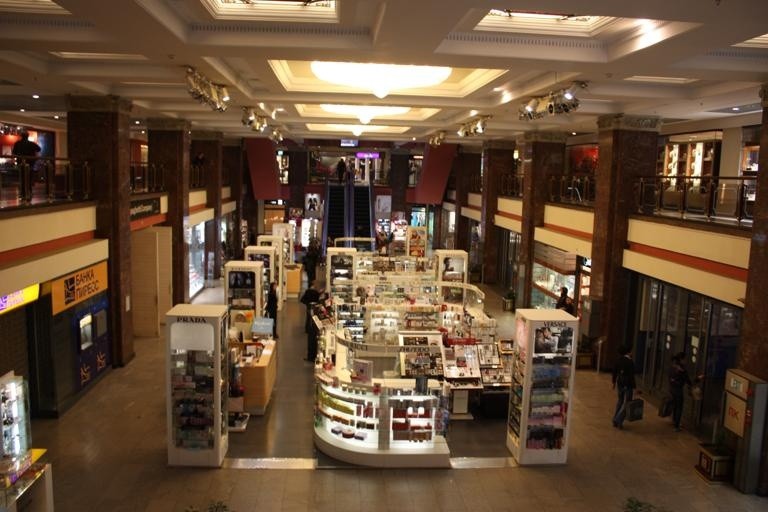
[658,397,675,417]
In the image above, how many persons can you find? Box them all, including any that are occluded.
[335,158,346,184]
[555,287,568,308]
[301,280,324,334]
[443,257,454,273]
[193,152,203,166]
[533,328,559,353]
[307,193,317,211]
[612,348,636,428]
[11,131,41,201]
[667,351,704,432]
[265,282,279,339]
[302,245,317,284]
[561,298,573,314]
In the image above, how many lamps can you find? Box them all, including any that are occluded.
[241,106,282,143]
[428,114,492,146]
[310,60,453,99]
[320,104,411,124]
[519,83,586,120]
[184,66,229,112]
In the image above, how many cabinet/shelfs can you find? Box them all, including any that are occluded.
[0,370,54,512]
[685,140,722,212]
[662,141,688,209]
[529,255,591,314]
[506,308,579,466]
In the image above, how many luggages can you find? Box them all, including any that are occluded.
[627,392,644,421]
